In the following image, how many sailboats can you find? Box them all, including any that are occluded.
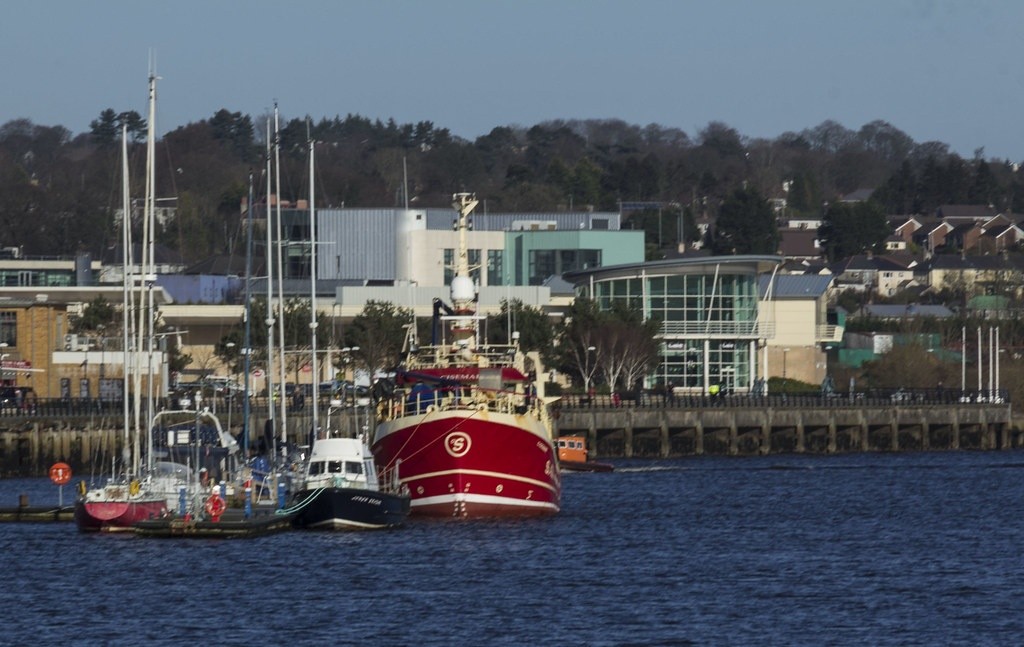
[79,55,415,539]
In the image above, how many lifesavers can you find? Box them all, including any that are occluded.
[426,404,439,413]
[476,402,489,411]
[50,462,72,483]
[207,495,225,516]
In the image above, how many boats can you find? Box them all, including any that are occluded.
[555,434,618,477]
[365,190,563,524]
